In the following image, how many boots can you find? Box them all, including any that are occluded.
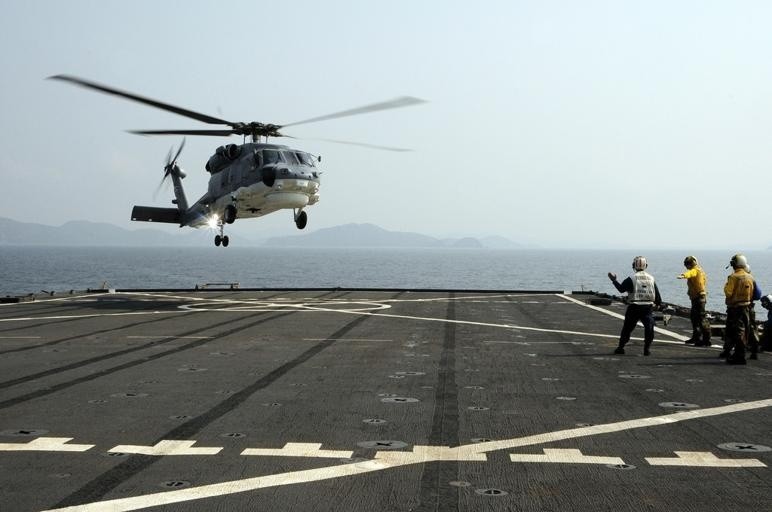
[615,343,625,354]
[644,341,651,356]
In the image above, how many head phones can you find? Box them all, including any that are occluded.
[632,256,648,268]
[762,296,768,308]
[692,256,698,265]
[730,254,739,267]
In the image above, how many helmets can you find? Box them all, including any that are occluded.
[632,256,647,271]
[730,254,751,273]
[761,295,772,309]
[684,256,697,269]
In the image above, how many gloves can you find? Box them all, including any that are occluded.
[608,272,616,281]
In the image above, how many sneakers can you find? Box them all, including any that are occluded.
[685,337,712,346]
[719,349,757,364]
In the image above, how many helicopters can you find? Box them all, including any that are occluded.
[45,74,429,247]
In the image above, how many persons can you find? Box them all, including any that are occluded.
[677,256,712,347]
[719,264,761,360]
[724,254,754,365]
[759,295,772,352]
[608,256,661,356]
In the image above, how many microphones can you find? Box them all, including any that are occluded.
[725,264,730,268]
